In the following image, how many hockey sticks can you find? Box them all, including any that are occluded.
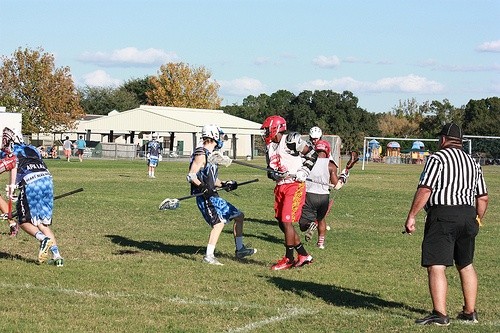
[210,151,334,188]
[335,150,360,192]
[158,179,256,209]
[0,187,83,219]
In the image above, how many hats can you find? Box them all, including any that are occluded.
[435,122,462,139]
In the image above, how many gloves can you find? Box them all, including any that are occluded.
[221,180,237,192]
[199,183,212,197]
[338,169,349,183]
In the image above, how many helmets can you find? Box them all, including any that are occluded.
[315,139,331,158]
[309,126,323,139]
[260,115,287,144]
[286,132,302,152]
[201,123,224,151]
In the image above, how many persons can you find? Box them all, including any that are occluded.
[0,126,63,267]
[186,124,257,265]
[306,126,334,159]
[137,143,141,156]
[176,143,180,155]
[298,140,350,249]
[64,136,74,162]
[405,123,489,326]
[76,136,86,163]
[147,134,162,178]
[260,115,318,270]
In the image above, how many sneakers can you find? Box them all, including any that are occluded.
[203,256,225,266]
[271,256,296,270]
[10,222,19,238]
[455,306,479,324]
[315,242,324,250]
[235,244,257,259]
[304,221,318,242]
[415,313,450,326]
[295,253,313,267]
[55,257,64,267]
[37,237,52,263]
